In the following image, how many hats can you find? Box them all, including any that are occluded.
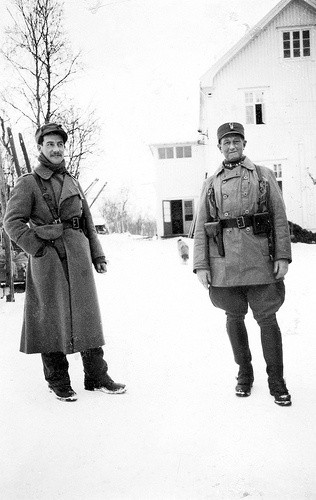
[35,123,68,145]
[217,122,244,143]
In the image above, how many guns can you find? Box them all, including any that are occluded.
[204,222,225,256]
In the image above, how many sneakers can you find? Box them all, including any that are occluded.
[84,375,127,394]
[235,374,253,396]
[270,384,292,404]
[48,382,77,401]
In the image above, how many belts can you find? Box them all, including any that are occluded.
[220,216,254,229]
[63,218,80,229]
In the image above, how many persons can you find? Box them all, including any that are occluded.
[193,123,292,406]
[2,124,127,401]
